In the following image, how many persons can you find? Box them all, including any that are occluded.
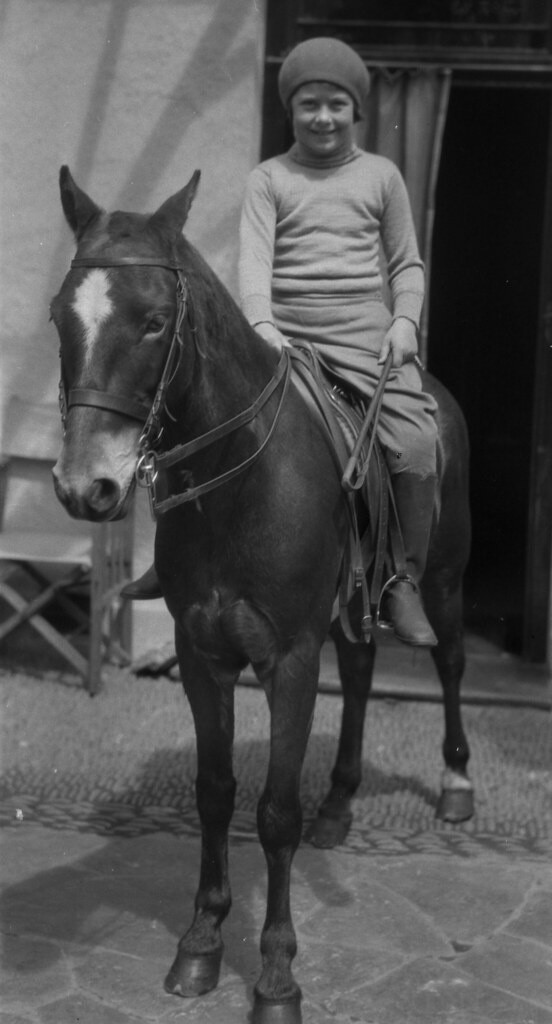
[115,38,446,652]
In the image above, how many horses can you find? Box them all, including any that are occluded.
[48,162,477,1023]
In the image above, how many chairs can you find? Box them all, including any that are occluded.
[1,481,138,697]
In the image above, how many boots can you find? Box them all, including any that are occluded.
[118,560,166,599]
[385,471,441,648]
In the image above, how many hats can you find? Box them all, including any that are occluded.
[280,36,370,122]
